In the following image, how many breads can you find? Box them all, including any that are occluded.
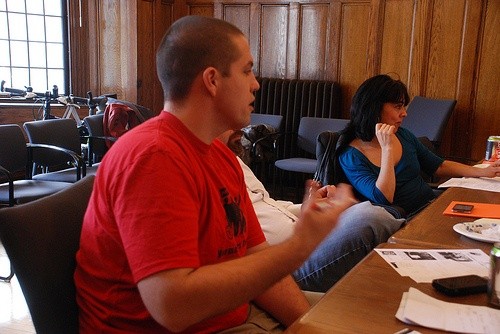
[462,218,500,239]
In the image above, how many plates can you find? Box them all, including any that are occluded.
[453,222,500,243]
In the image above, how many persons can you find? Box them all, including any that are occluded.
[215,130,407,293]
[336,74,500,215]
[74,15,351,334]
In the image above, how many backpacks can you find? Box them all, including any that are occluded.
[102,101,139,149]
[243,124,276,162]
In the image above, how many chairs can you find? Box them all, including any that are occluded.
[240,111,284,196]
[83,112,119,168]
[0,123,82,283]
[399,94,459,185]
[23,118,98,183]
[0,173,96,334]
[274,115,351,202]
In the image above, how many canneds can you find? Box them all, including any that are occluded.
[488,243,500,310]
[485,136,500,162]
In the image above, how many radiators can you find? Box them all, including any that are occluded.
[252,74,340,188]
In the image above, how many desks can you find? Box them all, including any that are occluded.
[283,156,500,334]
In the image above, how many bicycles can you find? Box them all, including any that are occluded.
[0,80,109,179]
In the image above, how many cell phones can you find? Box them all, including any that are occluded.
[452,204,474,213]
[433,275,490,297]
